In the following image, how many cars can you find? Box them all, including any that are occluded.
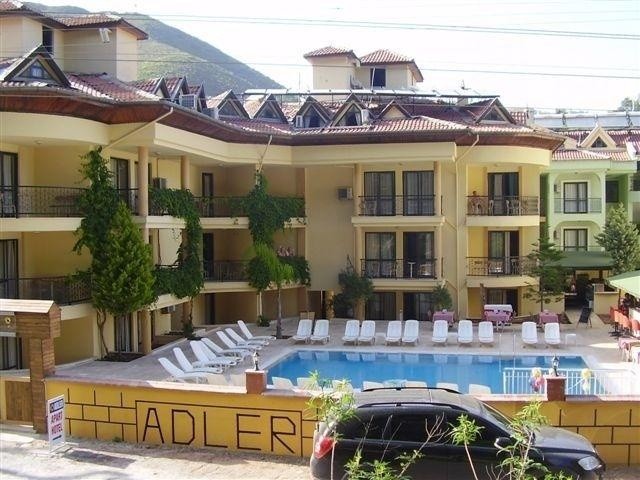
[309,388,606,480]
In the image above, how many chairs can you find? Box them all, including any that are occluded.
[427,309,559,331]
[468,198,523,216]
[511,258,520,275]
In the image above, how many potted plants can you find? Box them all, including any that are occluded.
[330,253,376,320]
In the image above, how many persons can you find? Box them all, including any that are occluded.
[471,191,484,215]
[570,278,576,293]
[277,246,294,256]
[622,293,640,308]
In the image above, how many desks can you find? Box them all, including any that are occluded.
[619,338,640,377]
